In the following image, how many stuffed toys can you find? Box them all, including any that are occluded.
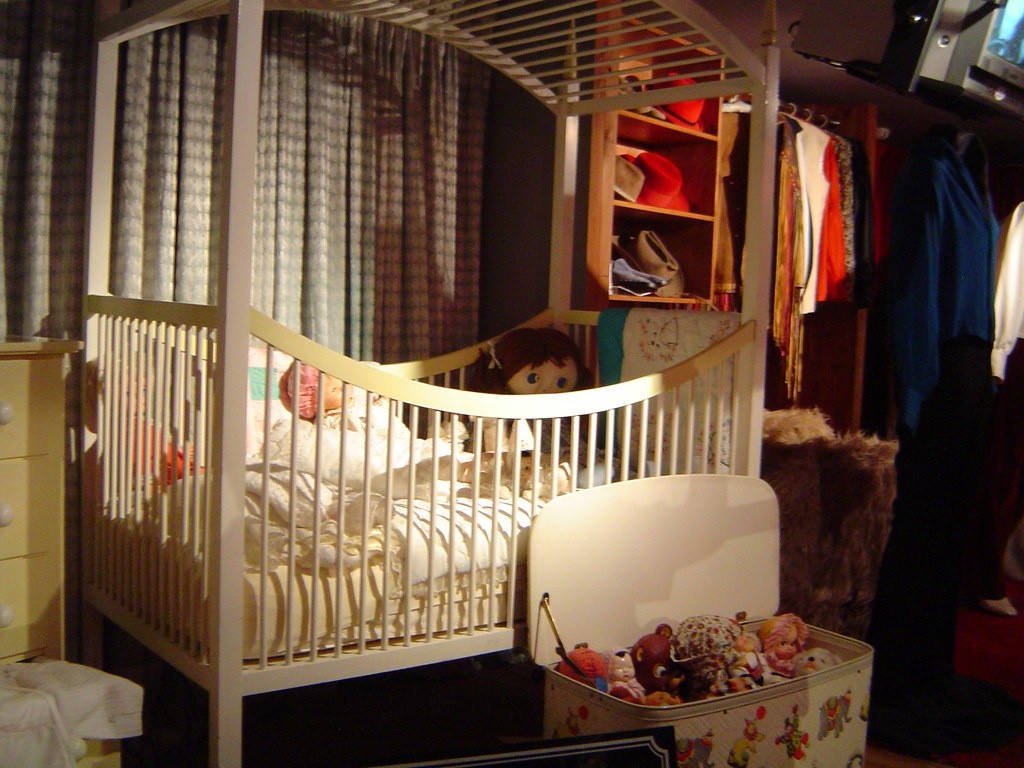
[469,325,598,465]
[100,358,210,495]
[285,353,373,441]
[559,606,852,708]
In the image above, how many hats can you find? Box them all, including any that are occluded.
[620,152,689,212]
[614,156,644,203]
[644,71,705,132]
[612,226,684,296]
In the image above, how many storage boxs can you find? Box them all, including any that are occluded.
[528,473,876,768]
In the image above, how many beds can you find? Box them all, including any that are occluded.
[81,0,782,768]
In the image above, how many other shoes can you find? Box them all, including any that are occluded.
[610,258,670,296]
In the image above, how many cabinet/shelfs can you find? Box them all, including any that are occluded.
[0,334,84,669]
[483,0,727,340]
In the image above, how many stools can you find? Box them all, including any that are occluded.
[0,657,144,768]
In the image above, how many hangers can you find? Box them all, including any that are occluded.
[722,93,829,129]
